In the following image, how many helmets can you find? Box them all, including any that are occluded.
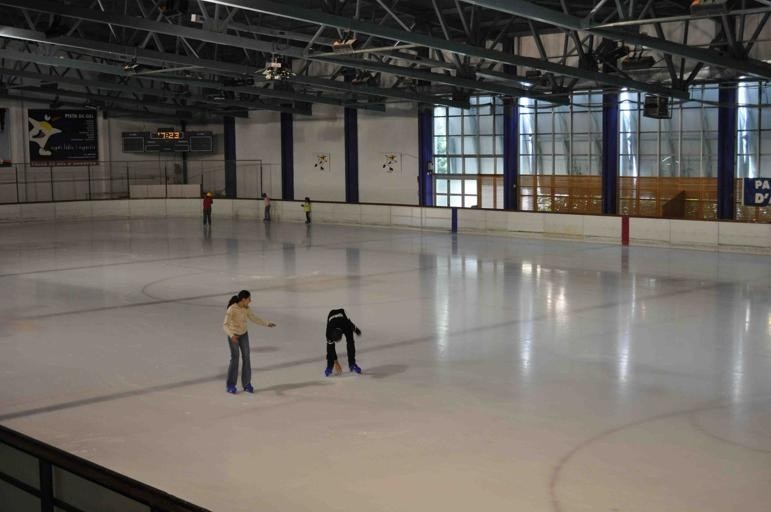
[206,192,212,197]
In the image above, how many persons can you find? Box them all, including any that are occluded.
[325,308,362,375]
[261,193,271,220]
[202,192,213,224]
[224,290,275,392]
[301,197,312,224]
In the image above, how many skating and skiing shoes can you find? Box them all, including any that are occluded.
[244,383,253,392]
[226,385,237,392]
[350,364,362,373]
[323,367,332,377]
